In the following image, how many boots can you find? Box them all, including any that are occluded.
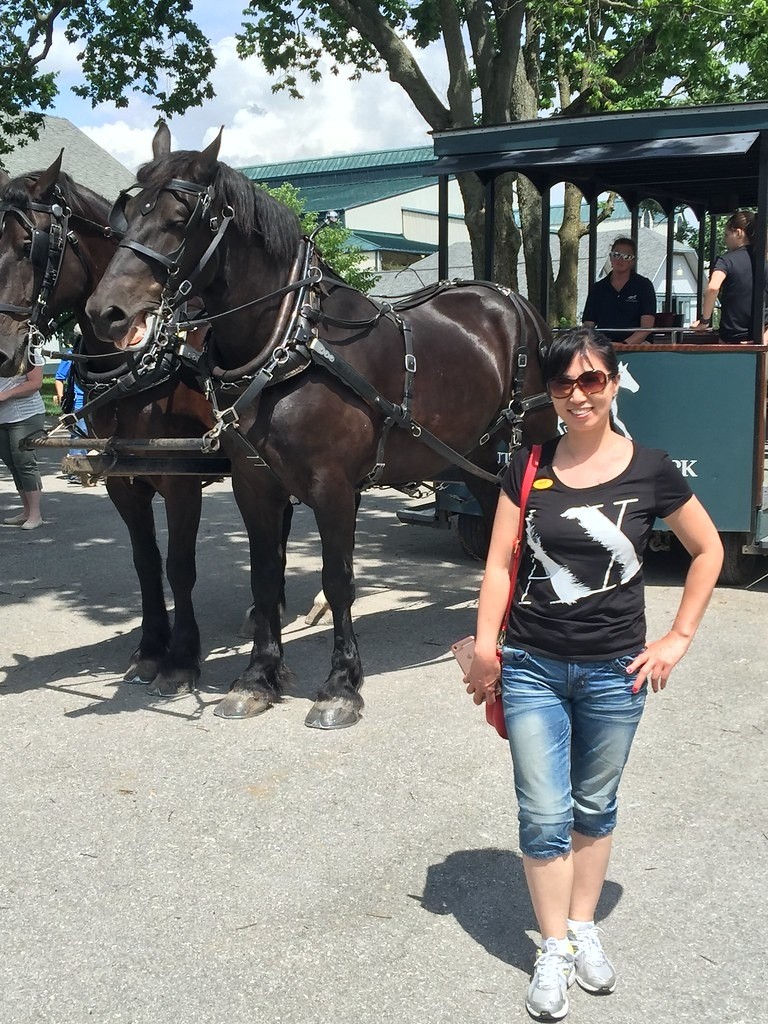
[21,490,43,529]
[4,489,29,525]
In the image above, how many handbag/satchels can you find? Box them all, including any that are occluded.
[60,371,76,414]
[485,646,509,740]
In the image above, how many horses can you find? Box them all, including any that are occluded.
[0,118,556,735]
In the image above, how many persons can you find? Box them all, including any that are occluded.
[690,211,768,345]
[582,239,656,344]
[463,327,726,1022]
[0,352,47,530]
[55,346,88,483]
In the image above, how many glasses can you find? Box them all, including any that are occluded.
[546,370,619,399]
[611,251,636,260]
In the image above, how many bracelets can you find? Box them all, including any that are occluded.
[700,314,711,324]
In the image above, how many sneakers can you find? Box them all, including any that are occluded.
[525,937,580,1019]
[68,475,83,484]
[567,927,617,994]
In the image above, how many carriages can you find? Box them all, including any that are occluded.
[0,100,768,731]
